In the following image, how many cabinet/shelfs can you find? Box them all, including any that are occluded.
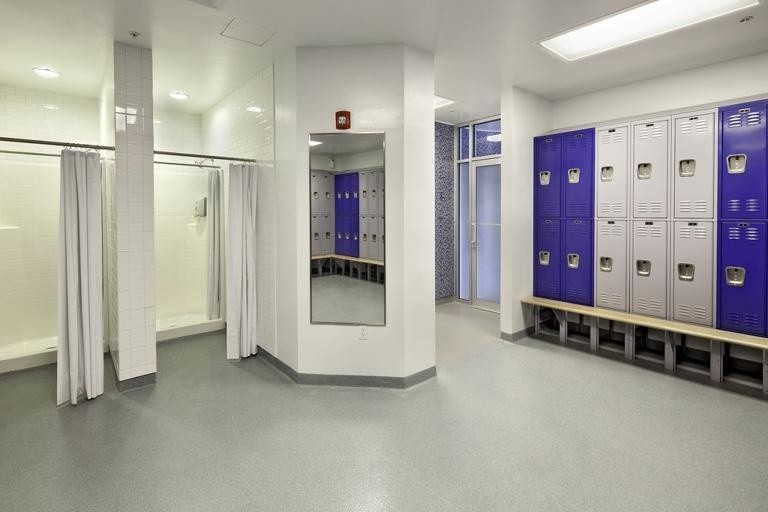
[631,114,669,321]
[309,167,384,266]
[669,106,719,328]
[563,124,597,308]
[718,101,766,340]
[531,131,563,302]
[594,121,631,312]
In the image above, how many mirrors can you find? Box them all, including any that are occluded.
[308,132,386,325]
